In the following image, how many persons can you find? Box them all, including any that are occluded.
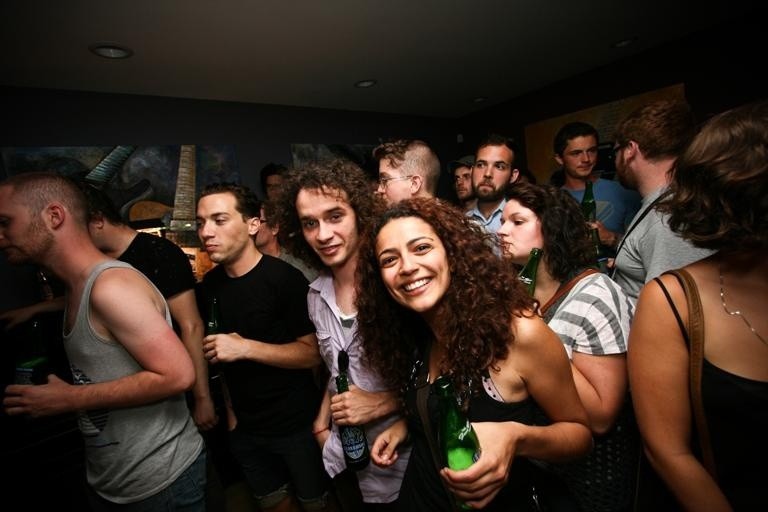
[554,83,768,511]
[359,138,592,511]
[193,181,328,510]
[2,173,226,509]
[501,185,634,512]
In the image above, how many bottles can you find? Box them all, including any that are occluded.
[514,248,544,297]
[202,297,220,379]
[15,321,50,423]
[589,228,609,276]
[579,179,596,222]
[436,375,487,512]
[335,374,370,470]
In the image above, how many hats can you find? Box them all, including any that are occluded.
[447,154,476,175]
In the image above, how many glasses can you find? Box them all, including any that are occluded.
[608,143,629,160]
[380,175,412,189]
